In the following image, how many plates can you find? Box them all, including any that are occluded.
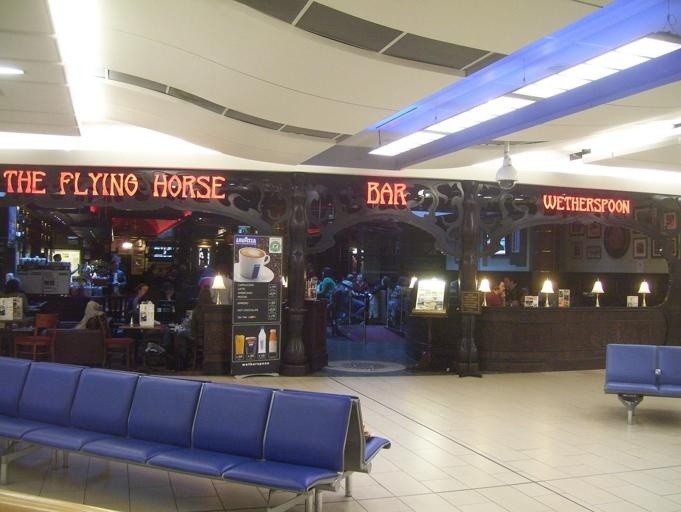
[233,263,274,282]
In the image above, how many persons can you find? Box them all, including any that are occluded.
[504,276,526,306]
[487,281,508,307]
[317,266,416,327]
[5,252,211,369]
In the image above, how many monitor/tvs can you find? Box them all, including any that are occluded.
[151,241,173,262]
[485,233,507,256]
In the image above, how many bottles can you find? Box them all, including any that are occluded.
[268,328,277,358]
[256,326,266,360]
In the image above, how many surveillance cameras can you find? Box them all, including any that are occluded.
[495,163,520,191]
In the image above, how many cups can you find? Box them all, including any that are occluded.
[234,333,244,360]
[245,337,256,360]
[237,247,270,279]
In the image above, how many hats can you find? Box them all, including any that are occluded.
[320,267,333,274]
[347,273,353,279]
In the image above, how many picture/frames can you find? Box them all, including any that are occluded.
[568,206,679,260]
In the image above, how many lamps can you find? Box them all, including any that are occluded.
[211,272,226,304]
[637,280,652,307]
[541,276,555,307]
[591,277,605,307]
[478,278,491,306]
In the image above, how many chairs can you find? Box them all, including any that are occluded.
[13,294,204,369]
[326,290,344,337]
[604,343,681,425]
